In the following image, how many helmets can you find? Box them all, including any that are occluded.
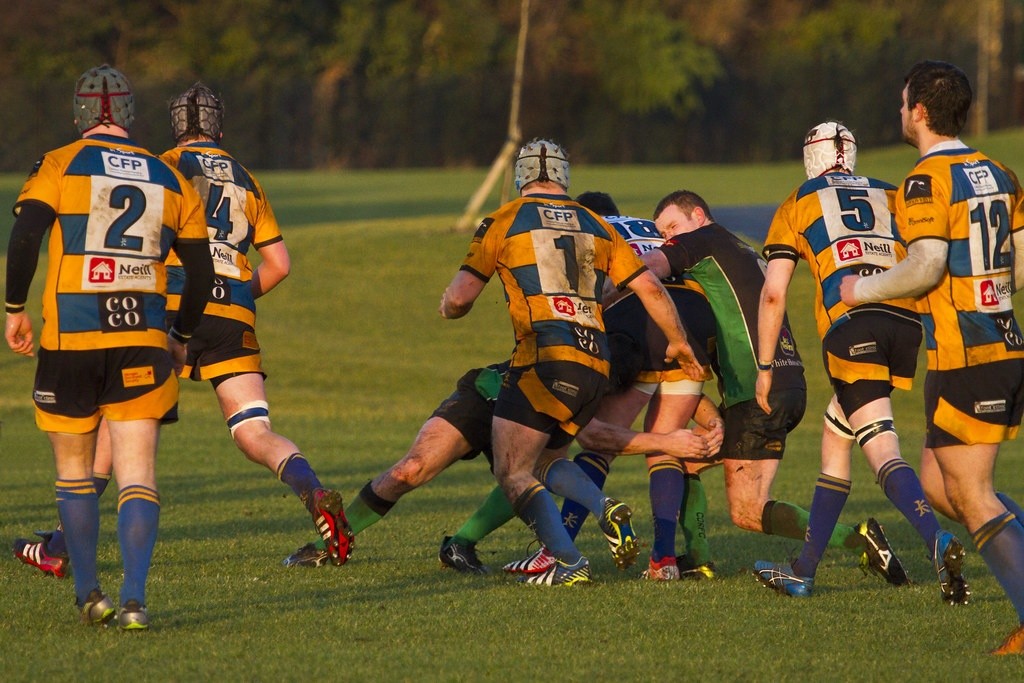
[71,64,137,133]
[170,82,226,144]
[514,135,571,191]
[803,122,857,181]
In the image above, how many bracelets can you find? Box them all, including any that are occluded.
[758,360,775,376]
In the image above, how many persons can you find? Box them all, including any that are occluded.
[753,119,974,607]
[13,83,354,578]
[839,59,1023,659]
[4,67,215,631]
[501,191,722,583]
[438,137,705,590]
[282,355,725,575]
[599,189,911,588]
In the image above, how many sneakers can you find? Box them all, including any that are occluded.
[634,556,680,582]
[118,598,148,631]
[514,555,591,589]
[501,545,556,573]
[854,517,908,587]
[752,560,815,600]
[14,531,69,579]
[602,497,641,572]
[675,554,717,580]
[439,536,491,578]
[926,530,971,607]
[987,624,1023,656]
[282,543,329,568]
[313,489,354,567]
[83,590,117,629]
